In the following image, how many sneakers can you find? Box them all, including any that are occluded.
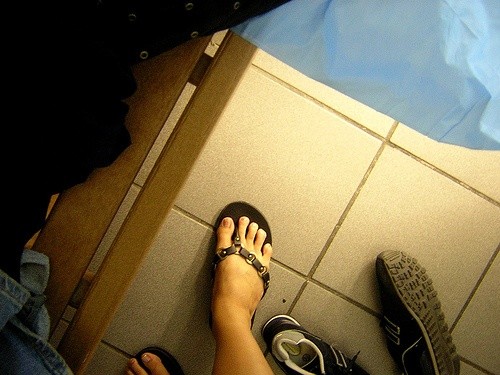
[260,311,372,375]
[375,250,462,375]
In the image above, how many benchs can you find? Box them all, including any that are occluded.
[16,20,260,374]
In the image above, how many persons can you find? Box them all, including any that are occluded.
[121,217,277,375]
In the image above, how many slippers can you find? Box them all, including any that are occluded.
[124,347,184,375]
[209,199,272,336]
